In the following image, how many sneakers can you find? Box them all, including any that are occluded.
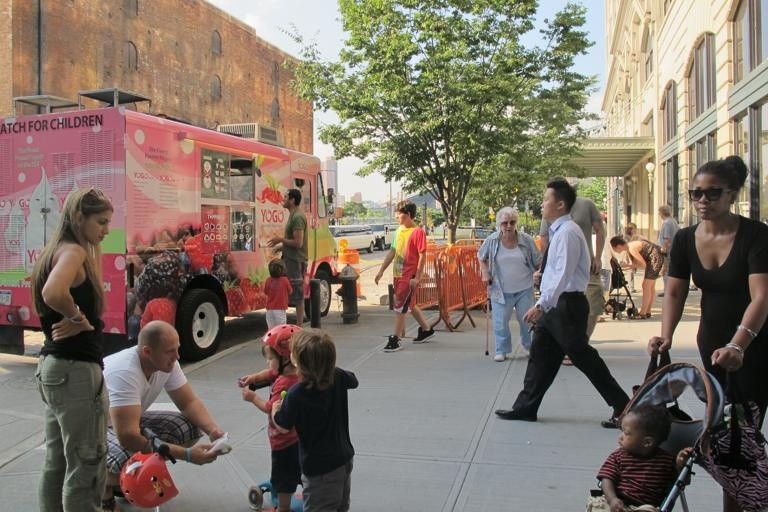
[383,334,404,352]
[411,325,436,343]
[494,352,506,361]
[518,338,531,356]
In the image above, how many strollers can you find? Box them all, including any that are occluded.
[580,342,730,512]
[605,253,642,322]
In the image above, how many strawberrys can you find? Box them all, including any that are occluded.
[238,263,269,312]
[222,280,250,317]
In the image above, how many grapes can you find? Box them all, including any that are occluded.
[126,290,141,337]
[212,252,237,284]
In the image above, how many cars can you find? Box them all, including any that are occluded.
[328,223,376,254]
[369,223,400,251]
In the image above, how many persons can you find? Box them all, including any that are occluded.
[589,408,694,511]
[238,190,357,511]
[101,320,223,510]
[374,201,436,353]
[648,157,768,510]
[495,180,630,429]
[35,186,113,511]
[594,205,678,322]
[477,207,540,361]
[539,177,606,366]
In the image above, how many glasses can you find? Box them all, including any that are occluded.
[500,219,517,227]
[688,187,738,203]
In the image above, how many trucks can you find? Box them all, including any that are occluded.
[0,87,340,361]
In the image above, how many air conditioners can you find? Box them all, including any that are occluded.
[216,122,280,146]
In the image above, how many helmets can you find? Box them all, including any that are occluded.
[119,449,180,509]
[258,324,306,360]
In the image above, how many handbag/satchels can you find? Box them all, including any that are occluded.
[692,361,768,512]
[631,342,695,422]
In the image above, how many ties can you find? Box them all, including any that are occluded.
[533,239,552,292]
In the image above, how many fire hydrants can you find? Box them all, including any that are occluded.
[336,264,360,323]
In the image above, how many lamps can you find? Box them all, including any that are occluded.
[603,198,607,210]
[618,176,638,201]
[645,162,655,193]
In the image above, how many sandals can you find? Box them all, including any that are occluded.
[628,311,651,320]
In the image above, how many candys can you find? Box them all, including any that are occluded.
[281,390,287,402]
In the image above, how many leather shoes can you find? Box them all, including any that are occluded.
[495,409,537,422]
[600,408,622,428]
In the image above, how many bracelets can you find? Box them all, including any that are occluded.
[725,342,745,361]
[67,305,80,322]
[70,313,84,326]
[186,448,193,464]
[736,325,758,339]
